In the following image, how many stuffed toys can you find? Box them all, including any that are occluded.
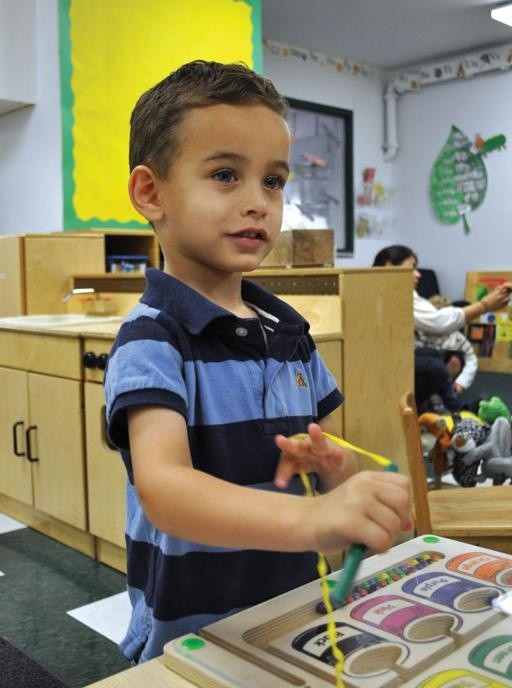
[417,395,511,487]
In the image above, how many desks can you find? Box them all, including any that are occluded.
[84,532,511,686]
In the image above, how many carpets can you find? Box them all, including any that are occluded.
[0,527,136,686]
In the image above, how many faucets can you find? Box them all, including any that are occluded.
[62,288,100,303]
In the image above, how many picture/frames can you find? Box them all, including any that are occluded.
[266,96,359,259]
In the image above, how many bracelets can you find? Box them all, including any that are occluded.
[478,300,489,313]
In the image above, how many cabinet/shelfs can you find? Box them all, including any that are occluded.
[1,230,158,316]
[81,323,344,575]
[2,323,91,552]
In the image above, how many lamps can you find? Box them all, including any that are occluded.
[484,1,512,33]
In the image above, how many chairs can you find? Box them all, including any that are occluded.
[400,390,511,553]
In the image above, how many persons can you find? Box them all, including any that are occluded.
[415,295,481,395]
[101,57,414,666]
[369,243,510,410]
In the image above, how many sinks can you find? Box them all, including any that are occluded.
[0,313,123,330]
